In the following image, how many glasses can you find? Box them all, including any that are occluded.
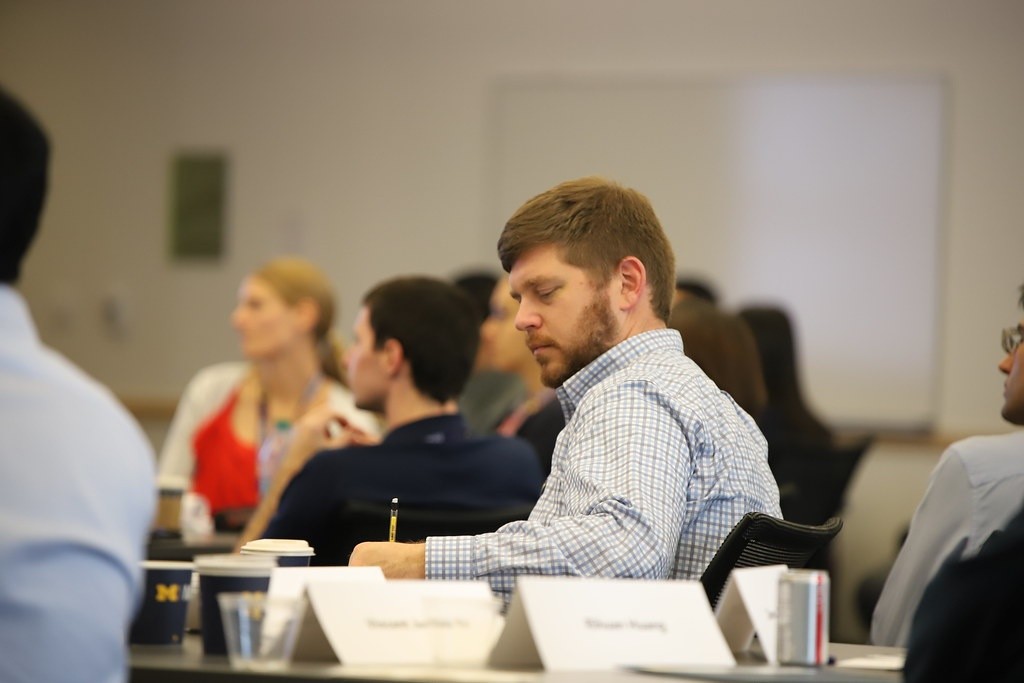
[1002,327,1024,354]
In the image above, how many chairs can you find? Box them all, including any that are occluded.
[700,511,843,612]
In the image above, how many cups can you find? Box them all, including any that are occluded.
[197,552,273,657]
[218,592,310,671]
[241,536,316,565]
[122,559,193,644]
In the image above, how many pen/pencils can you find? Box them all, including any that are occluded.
[388,497,398,542]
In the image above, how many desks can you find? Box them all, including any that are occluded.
[130,631,904,683]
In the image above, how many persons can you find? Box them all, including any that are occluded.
[156,258,381,533]
[868,283,1024,648]
[0,82,159,682]
[348,176,784,617]
[455,266,843,519]
[233,275,541,565]
